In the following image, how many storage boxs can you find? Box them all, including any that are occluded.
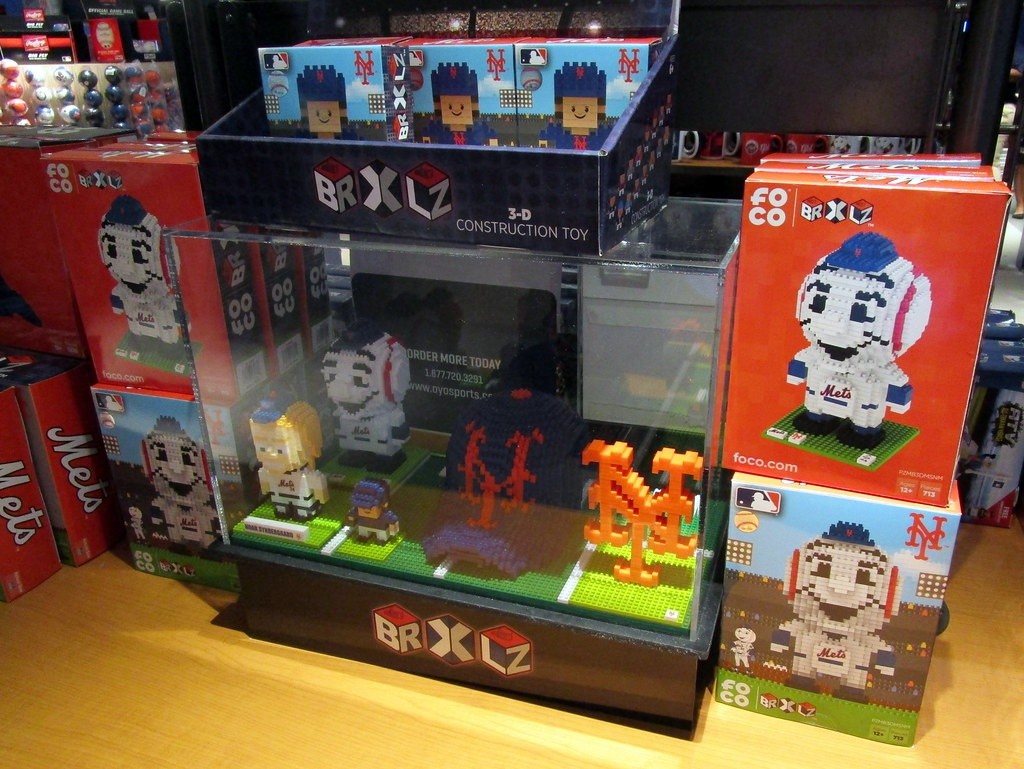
[1,0,682,606]
[160,198,742,742]
[671,130,1024,749]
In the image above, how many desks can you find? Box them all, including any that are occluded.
[671,155,755,179]
